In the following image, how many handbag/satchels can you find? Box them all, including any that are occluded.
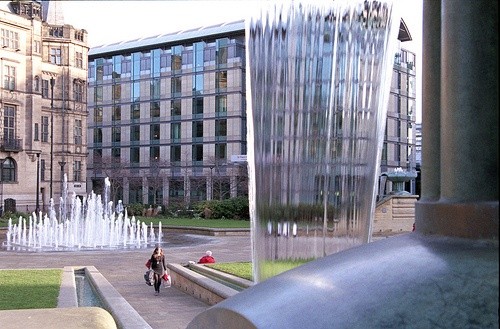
[144,269,155,286]
[146,259,152,269]
[162,270,171,288]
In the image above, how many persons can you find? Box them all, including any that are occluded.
[199,250,216,263]
[150,247,168,296]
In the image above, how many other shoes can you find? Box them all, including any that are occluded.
[154,290,159,295]
